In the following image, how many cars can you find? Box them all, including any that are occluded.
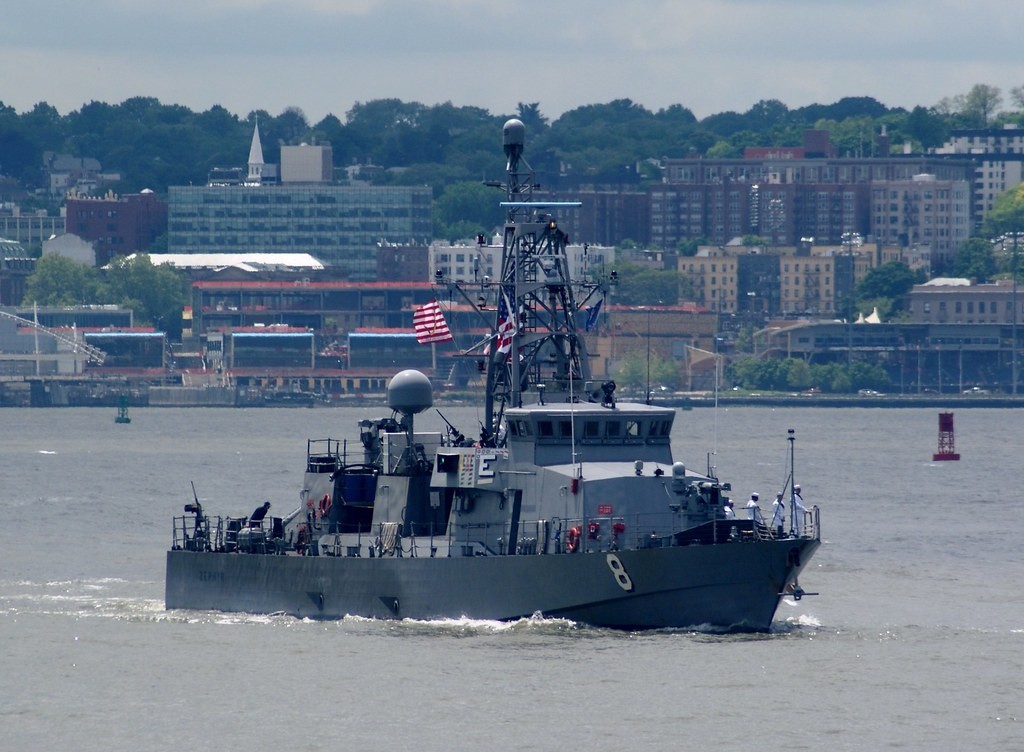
[859,389,878,397]
[964,386,987,395]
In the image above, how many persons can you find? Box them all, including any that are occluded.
[772,492,786,538]
[723,500,736,519]
[747,493,761,527]
[789,485,811,538]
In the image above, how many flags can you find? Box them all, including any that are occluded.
[485,284,525,362]
[413,296,453,344]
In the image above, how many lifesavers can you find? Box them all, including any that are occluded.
[567,528,579,551]
[321,493,330,515]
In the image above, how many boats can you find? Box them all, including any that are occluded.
[115,394,131,423]
[167,116,822,636]
